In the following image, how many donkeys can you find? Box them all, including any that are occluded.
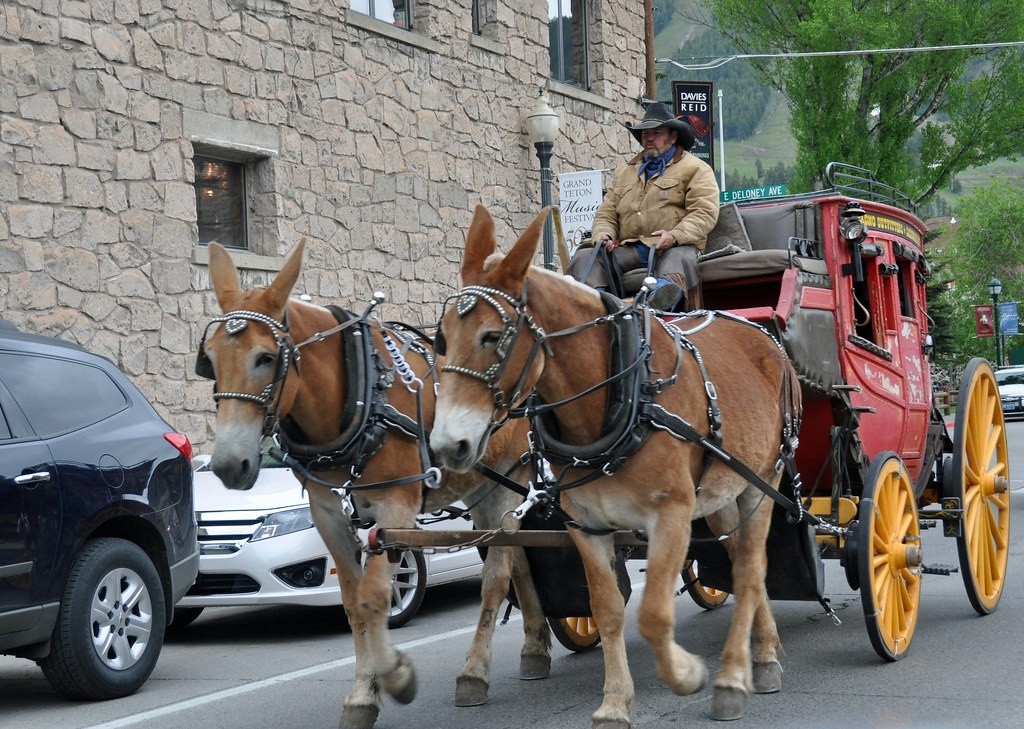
[198,237,554,729]
[425,206,800,729]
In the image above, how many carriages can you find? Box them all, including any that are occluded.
[204,162,1009,729]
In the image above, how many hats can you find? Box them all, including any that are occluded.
[625,103,695,150]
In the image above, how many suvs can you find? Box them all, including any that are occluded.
[0,315,200,700]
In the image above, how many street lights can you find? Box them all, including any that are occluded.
[989,272,1002,368]
[527,86,560,271]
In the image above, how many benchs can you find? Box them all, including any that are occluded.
[621,199,829,296]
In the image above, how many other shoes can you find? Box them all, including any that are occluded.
[647,283,681,311]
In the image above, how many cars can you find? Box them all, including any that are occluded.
[167,434,485,631]
[994,368,1024,420]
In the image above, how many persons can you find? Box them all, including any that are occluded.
[567,103,720,312]
